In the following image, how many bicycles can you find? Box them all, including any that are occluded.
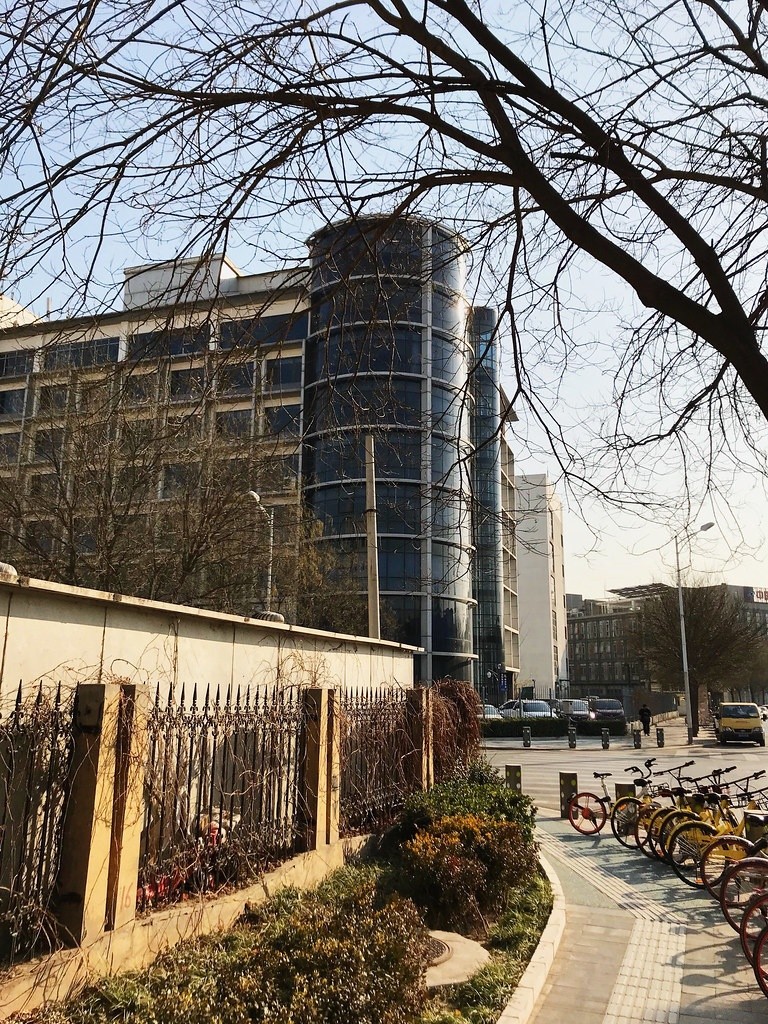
[568,759,768,1002]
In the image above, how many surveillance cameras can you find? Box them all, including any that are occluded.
[487,672,491,678]
[498,667,500,670]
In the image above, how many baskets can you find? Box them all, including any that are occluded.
[707,786,728,797]
[647,781,669,798]
[728,793,749,808]
[757,796,768,815]
[685,785,703,797]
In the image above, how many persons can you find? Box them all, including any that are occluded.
[639,704,652,736]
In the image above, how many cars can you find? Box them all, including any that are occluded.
[495,695,600,719]
[476,705,503,720]
[711,701,768,747]
[587,699,625,721]
[511,701,558,721]
[560,699,591,723]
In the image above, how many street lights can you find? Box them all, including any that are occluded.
[246,490,273,612]
[487,669,500,710]
[675,522,714,746]
